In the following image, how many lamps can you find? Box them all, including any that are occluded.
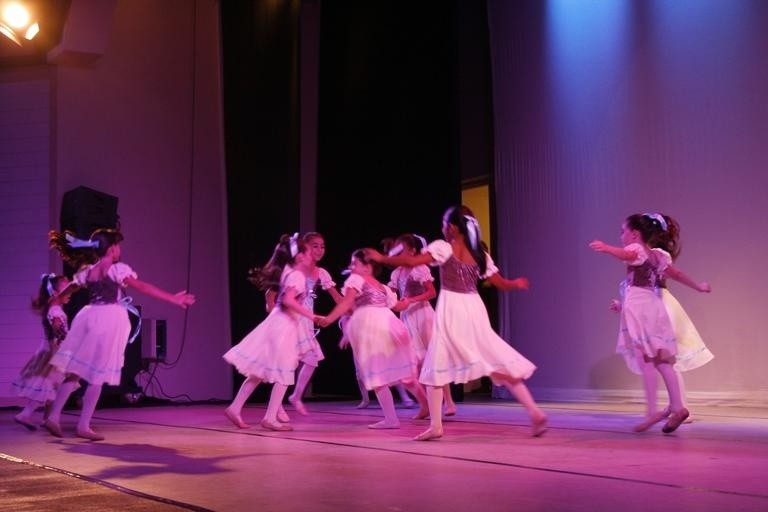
[0,0,39,49]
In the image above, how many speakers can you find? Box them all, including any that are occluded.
[141,319,167,362]
[61,185,119,242]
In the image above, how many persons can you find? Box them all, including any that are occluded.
[43,228,196,440]
[223,204,546,440]
[609,213,715,423]
[589,213,712,433]
[10,273,71,432]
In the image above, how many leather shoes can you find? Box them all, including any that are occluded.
[288,395,308,416]
[44,419,65,439]
[277,406,290,423]
[15,412,40,431]
[661,409,689,433]
[260,418,294,432]
[412,429,443,442]
[224,408,251,429]
[75,425,105,441]
[631,411,662,432]
[367,420,399,429]
[412,408,430,420]
[444,404,456,418]
[530,413,548,439]
